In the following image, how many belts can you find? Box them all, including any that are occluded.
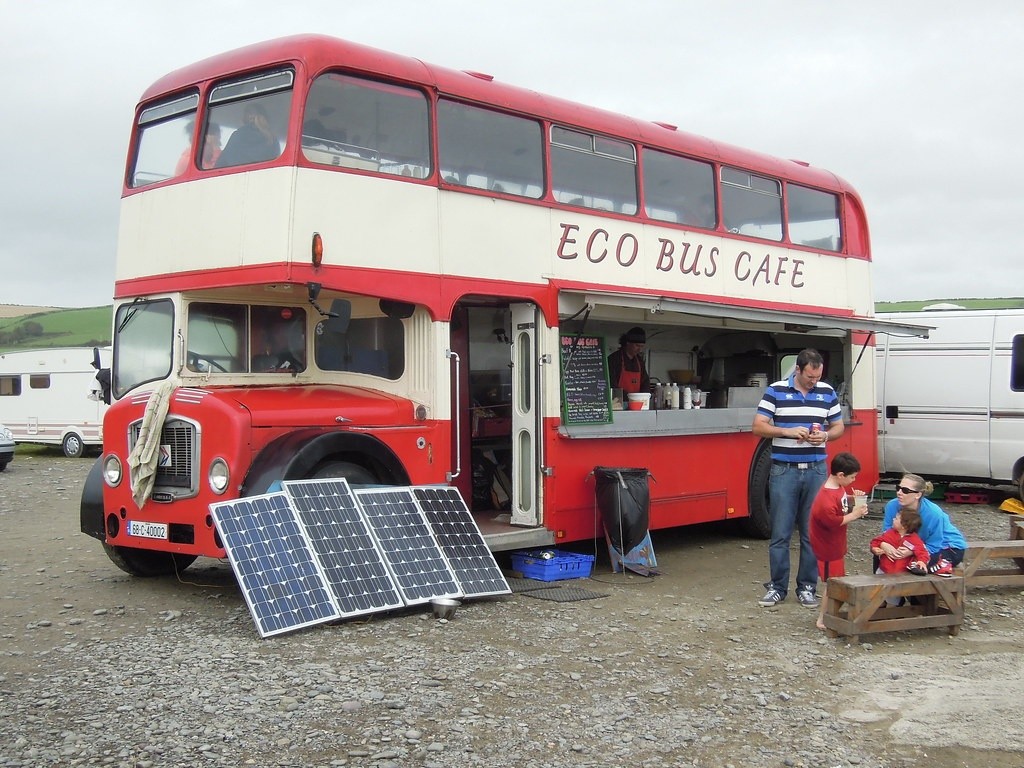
[771,458,824,470]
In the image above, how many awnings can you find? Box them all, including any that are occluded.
[552,278,937,385]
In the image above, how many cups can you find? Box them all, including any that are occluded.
[610,387,623,410]
[854,495,868,507]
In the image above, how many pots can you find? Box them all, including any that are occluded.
[746,372,768,387]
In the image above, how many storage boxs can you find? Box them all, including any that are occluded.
[511,550,594,583]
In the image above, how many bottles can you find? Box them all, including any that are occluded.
[654,383,701,409]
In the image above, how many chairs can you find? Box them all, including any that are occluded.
[320,335,389,372]
[244,349,299,369]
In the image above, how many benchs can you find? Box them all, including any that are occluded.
[953,540,1024,598]
[822,575,966,646]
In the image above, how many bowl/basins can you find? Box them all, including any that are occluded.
[669,369,695,384]
[623,401,644,410]
[429,598,462,620]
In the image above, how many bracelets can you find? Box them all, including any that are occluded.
[825,431,828,442]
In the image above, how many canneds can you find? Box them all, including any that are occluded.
[809,423,821,435]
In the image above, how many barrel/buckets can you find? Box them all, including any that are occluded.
[627,392,651,410]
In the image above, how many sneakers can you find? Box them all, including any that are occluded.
[927,553,952,577]
[757,589,782,606]
[798,591,818,608]
[906,557,927,574]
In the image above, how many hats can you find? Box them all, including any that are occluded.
[628,327,646,344]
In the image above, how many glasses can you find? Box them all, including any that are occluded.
[896,484,919,494]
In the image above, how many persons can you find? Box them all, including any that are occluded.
[753,349,844,608]
[869,508,931,608]
[214,104,281,169]
[810,452,869,630]
[873,473,967,607]
[608,327,650,402]
[175,123,221,176]
